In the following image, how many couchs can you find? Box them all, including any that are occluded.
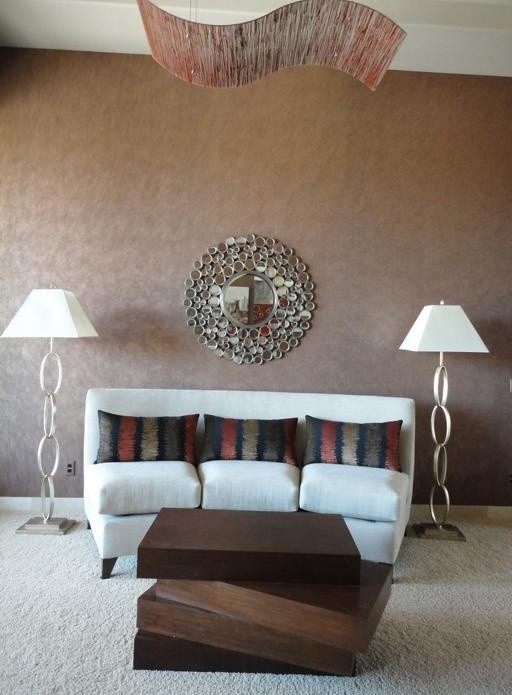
[84,383,417,579]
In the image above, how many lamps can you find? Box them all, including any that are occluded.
[396,300,494,543]
[1,282,101,539]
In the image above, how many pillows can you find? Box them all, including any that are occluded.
[93,409,201,466]
[201,413,299,466]
[302,414,403,474]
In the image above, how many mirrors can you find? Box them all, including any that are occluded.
[216,267,280,331]
